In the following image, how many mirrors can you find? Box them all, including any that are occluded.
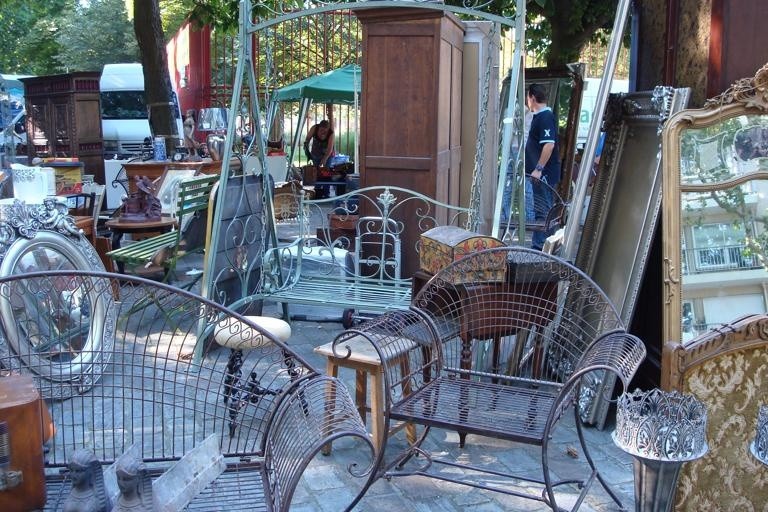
[659,61,768,351]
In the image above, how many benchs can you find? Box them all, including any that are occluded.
[183,183,475,374]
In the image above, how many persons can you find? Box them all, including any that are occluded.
[182,108,198,158]
[39,198,83,242]
[503,84,560,250]
[303,120,336,199]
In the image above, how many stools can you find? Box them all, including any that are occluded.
[313,329,418,464]
[214,315,311,437]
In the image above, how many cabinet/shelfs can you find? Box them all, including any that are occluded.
[411,268,559,448]
[16,72,108,210]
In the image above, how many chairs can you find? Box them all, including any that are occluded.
[0,265,376,510]
[1,168,361,336]
[330,243,651,511]
[495,171,570,251]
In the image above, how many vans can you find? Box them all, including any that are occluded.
[99,63,185,157]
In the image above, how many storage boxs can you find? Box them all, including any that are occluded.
[418,225,509,286]
[507,243,561,283]
